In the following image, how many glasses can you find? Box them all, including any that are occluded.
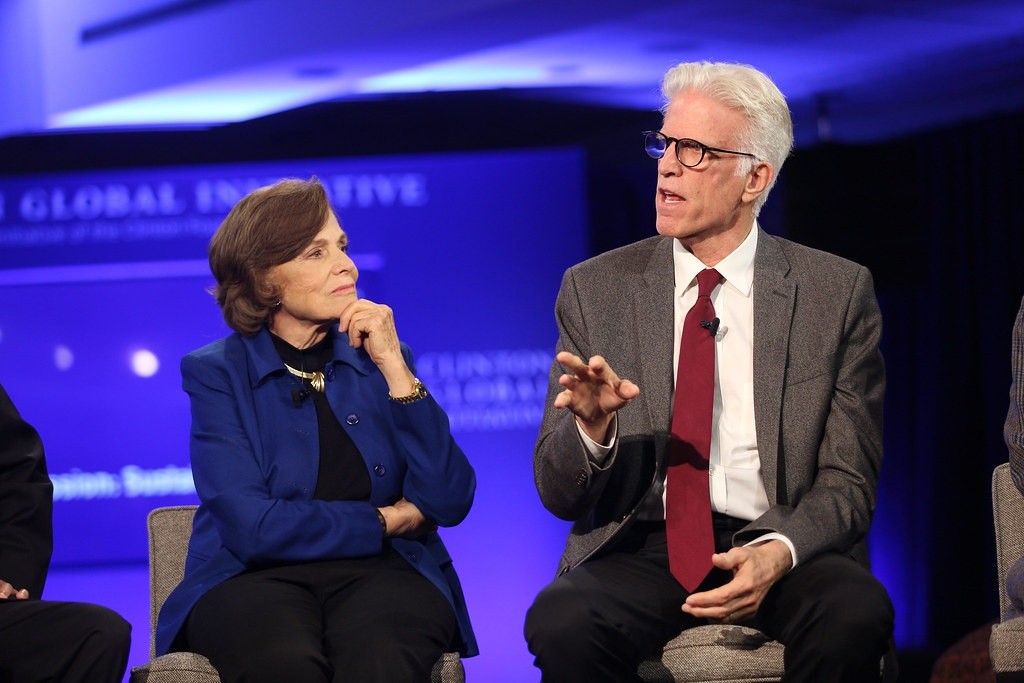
[641,129,761,167]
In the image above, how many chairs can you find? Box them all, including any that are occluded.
[145,505,467,683]
[989,462,1023,683]
[636,624,785,683]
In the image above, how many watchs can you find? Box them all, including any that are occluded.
[388,377,428,405]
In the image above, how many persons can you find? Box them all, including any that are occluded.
[155,175,480,683]
[1006,296,1024,617]
[523,59,896,683]
[0,383,133,683]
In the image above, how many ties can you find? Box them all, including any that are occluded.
[666,269,725,593]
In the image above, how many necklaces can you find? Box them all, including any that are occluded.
[283,361,325,392]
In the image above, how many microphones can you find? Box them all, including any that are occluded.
[699,317,721,336]
[290,387,314,409]
